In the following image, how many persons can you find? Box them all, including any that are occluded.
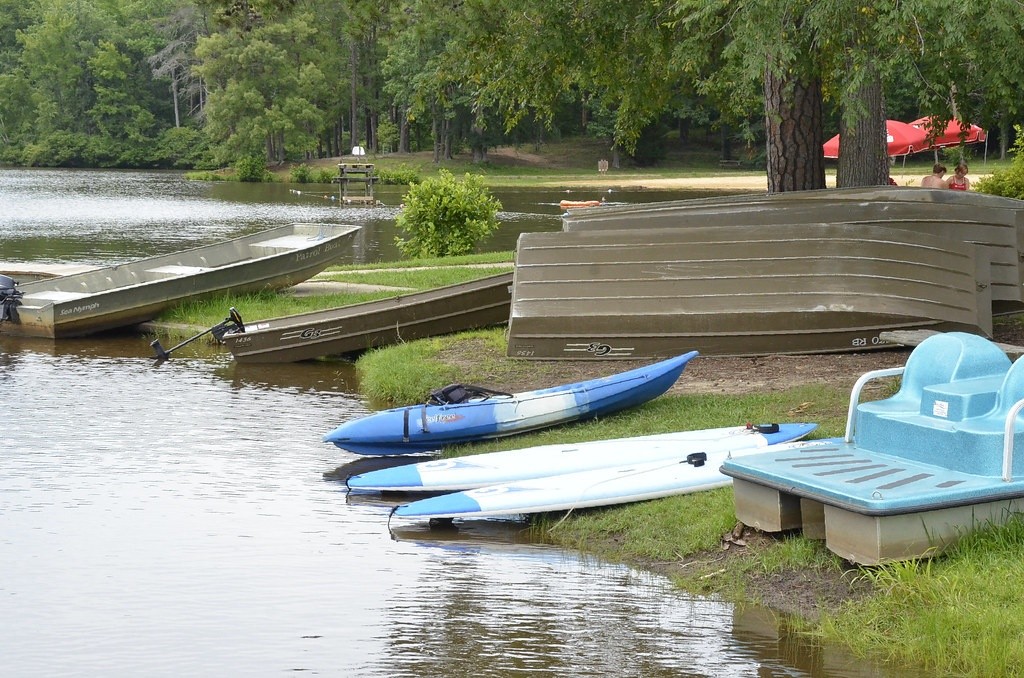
[947,163,970,191]
[922,163,948,190]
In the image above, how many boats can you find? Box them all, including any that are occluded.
[504,186,1023,359]
[720,330,1024,570]
[3,222,363,340]
[560,199,600,209]
[319,351,701,459]
[148,270,511,369]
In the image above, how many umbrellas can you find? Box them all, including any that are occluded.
[822,119,932,159]
[909,113,986,164]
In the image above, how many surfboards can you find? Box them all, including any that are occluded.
[391,437,845,525]
[345,422,819,496]
